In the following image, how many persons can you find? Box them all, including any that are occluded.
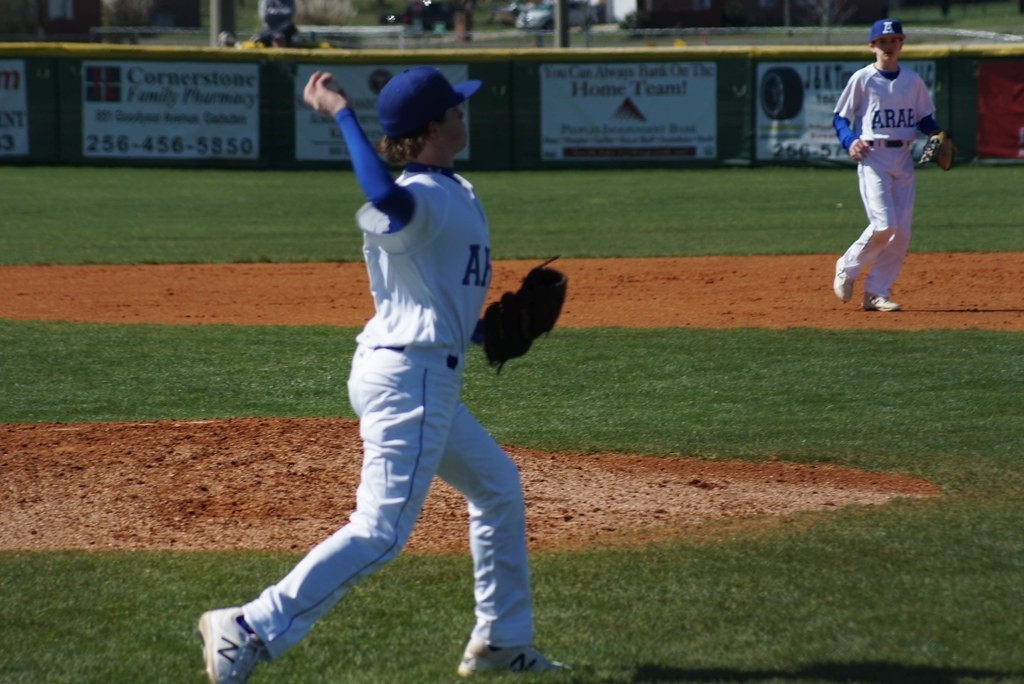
[197,67,569,684]
[833,18,947,311]
[254,0,298,48]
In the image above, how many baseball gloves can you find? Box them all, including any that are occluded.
[484,265,570,365]
[918,132,954,171]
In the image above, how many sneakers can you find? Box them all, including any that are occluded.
[864,294,899,312]
[457,638,571,676]
[833,257,853,303]
[198,607,263,684]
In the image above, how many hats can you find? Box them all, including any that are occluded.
[376,67,481,135]
[868,19,906,43]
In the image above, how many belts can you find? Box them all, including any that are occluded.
[375,346,458,371]
[868,140,910,148]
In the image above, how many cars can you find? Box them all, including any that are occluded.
[509,0,608,32]
[380,0,473,38]
